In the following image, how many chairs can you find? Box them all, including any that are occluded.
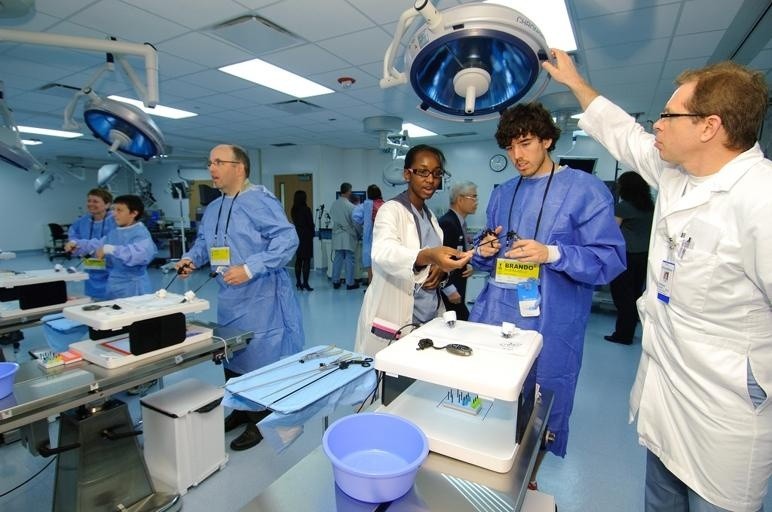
[49,223,71,261]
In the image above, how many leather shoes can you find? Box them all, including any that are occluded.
[346,283,360,289]
[230,422,262,450]
[224,408,249,432]
[604,335,632,345]
[333,283,341,289]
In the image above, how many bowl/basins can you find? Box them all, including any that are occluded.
[321,411,430,505]
[0,361,20,400]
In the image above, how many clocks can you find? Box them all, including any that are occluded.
[490,154,507,173]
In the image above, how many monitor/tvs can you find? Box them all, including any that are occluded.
[335,191,367,205]
[558,156,600,175]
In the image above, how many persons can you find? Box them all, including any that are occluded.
[67,195,155,296]
[329,183,361,290]
[290,191,316,290]
[174,144,305,451]
[351,184,387,284]
[435,181,477,323]
[357,144,477,412]
[541,47,770,511]
[599,171,655,344]
[467,104,627,489]
[65,189,116,295]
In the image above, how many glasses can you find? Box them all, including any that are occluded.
[461,193,479,201]
[406,168,445,178]
[660,111,698,120]
[207,159,241,168]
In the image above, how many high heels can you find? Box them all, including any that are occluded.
[296,282,302,289]
[302,284,314,291]
